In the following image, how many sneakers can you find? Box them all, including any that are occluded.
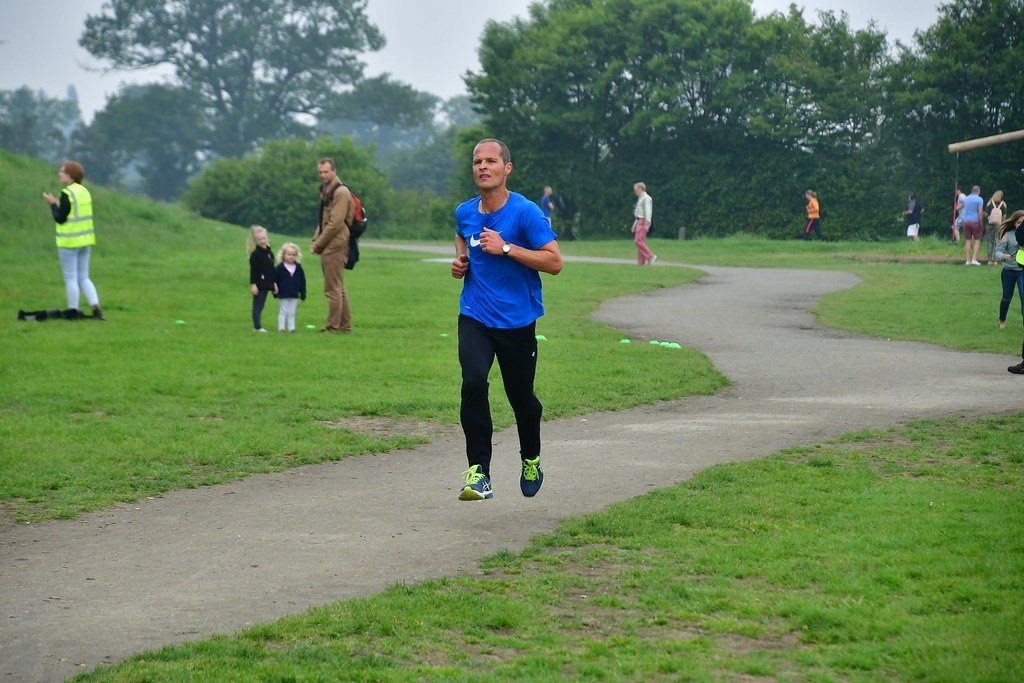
[458,463,493,501]
[519,456,544,498]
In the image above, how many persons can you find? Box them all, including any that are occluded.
[539,184,555,230]
[632,182,657,265]
[1007,218,1024,374]
[246,223,306,331]
[42,160,103,318]
[311,157,358,333]
[952,186,1006,267]
[902,191,925,240]
[994,210,1024,329]
[805,190,829,242]
[449,138,563,501]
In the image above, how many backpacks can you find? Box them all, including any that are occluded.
[988,200,1004,225]
[332,183,368,239]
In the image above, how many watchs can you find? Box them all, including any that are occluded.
[502,239,511,255]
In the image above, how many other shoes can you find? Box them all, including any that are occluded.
[988,261,993,266]
[994,261,998,265]
[648,255,656,265]
[972,260,981,266]
[999,321,1005,328]
[1008,360,1024,374]
[966,260,972,265]
